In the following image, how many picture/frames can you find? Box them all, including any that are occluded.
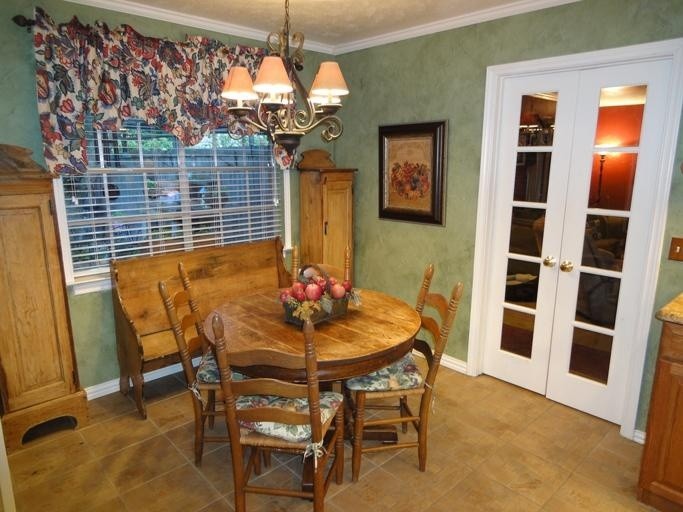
[378,120,448,227]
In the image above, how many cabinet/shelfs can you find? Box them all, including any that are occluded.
[635,323,683,512]
[0,144,90,457]
[297,149,358,287]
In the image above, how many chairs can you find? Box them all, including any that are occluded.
[534,217,620,323]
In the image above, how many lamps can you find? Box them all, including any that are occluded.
[595,144,613,202]
[220,1,350,171]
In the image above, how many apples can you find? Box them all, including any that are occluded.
[278,276,354,301]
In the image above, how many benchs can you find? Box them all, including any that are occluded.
[109,235,294,420]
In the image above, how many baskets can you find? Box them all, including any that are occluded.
[282,264,348,327]
